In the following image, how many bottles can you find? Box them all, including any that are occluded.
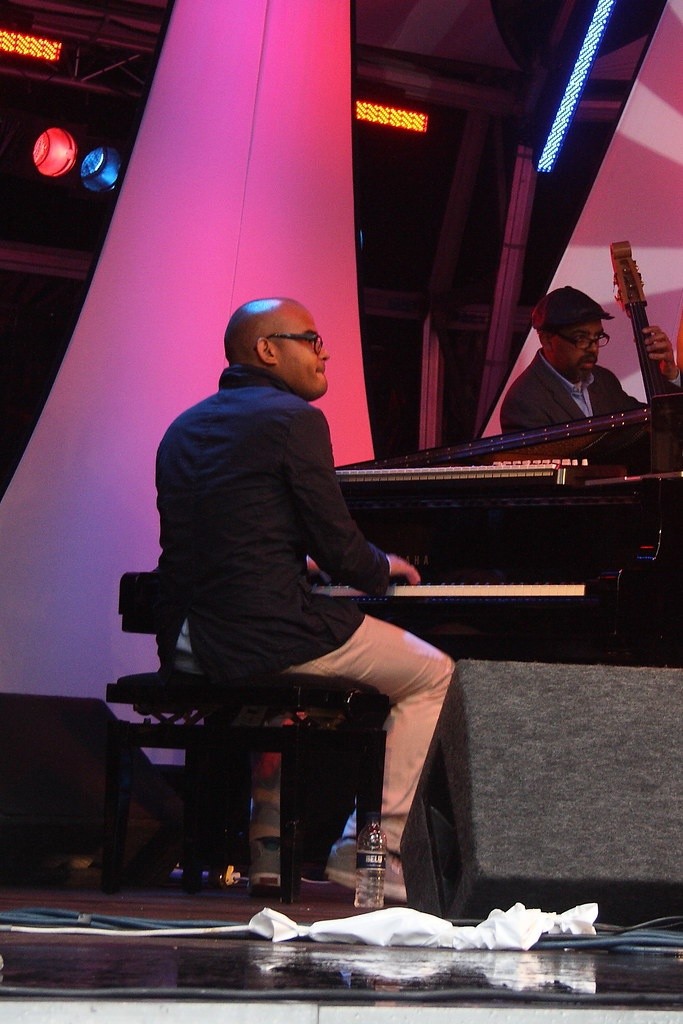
[354,811,387,909]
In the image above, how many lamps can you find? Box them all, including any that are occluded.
[13,117,124,193]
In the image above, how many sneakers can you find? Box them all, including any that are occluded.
[325,840,408,902]
[244,837,281,898]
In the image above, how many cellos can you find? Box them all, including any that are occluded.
[608,235,672,403]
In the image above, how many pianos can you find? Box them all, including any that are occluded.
[115,402,683,884]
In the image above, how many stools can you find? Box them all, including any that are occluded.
[99,671,391,911]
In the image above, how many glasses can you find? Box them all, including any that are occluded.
[552,330,612,351]
[254,332,322,356]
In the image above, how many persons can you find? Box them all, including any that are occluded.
[155,297,455,903]
[499,286,682,460]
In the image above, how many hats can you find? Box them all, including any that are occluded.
[532,286,615,331]
